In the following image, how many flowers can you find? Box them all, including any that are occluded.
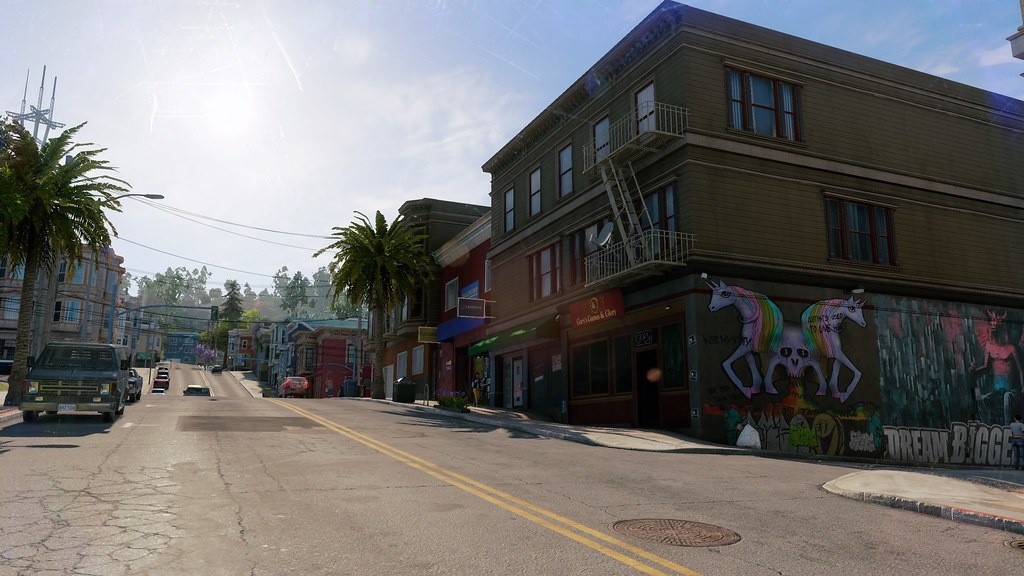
[435,388,469,408]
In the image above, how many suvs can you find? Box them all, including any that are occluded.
[18,342,130,422]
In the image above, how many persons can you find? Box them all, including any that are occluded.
[472,373,490,406]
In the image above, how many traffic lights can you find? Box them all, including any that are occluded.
[211,308,217,320]
[228,355,232,360]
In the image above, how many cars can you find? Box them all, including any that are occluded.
[159,366,169,370]
[156,375,169,379]
[157,370,169,375]
[278,377,310,398]
[208,366,213,370]
[159,362,170,367]
[150,388,166,396]
[153,379,169,390]
[121,370,143,403]
[183,384,211,397]
[211,365,222,373]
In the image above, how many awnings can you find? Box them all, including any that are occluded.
[467,311,559,357]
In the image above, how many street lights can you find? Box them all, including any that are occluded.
[79,193,164,341]
